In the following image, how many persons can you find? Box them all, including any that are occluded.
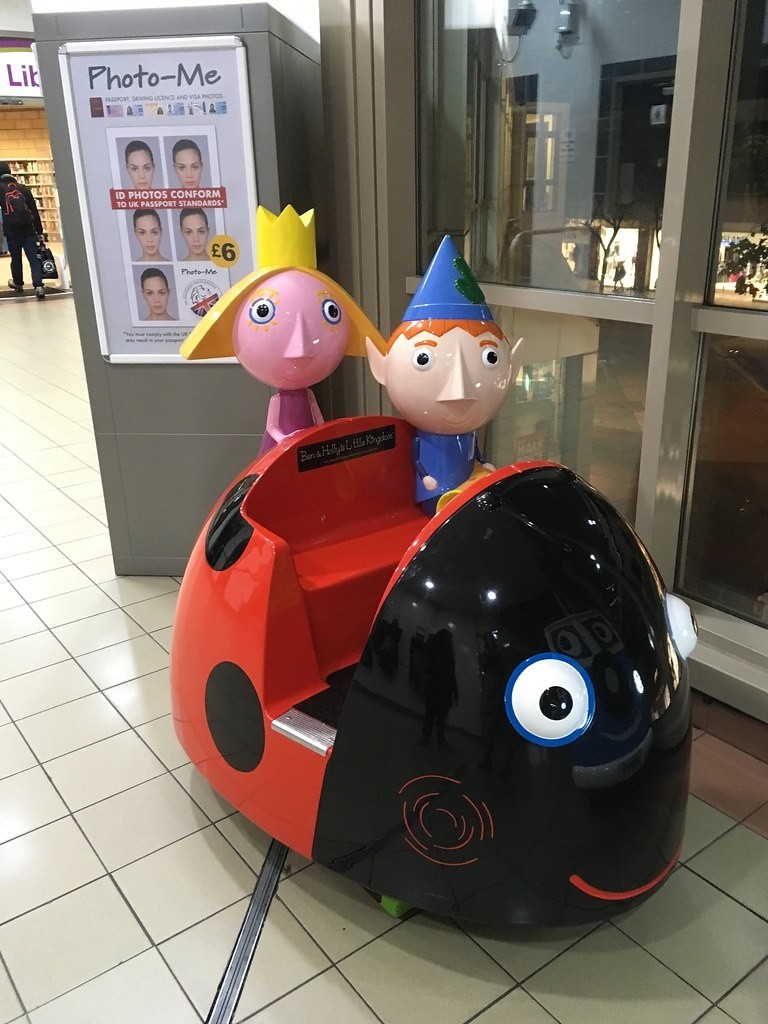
[180,208,211,261]
[133,209,169,261]
[366,234,524,518]
[180,205,388,459]
[0,162,45,298]
[125,141,155,189]
[141,268,177,321]
[612,260,626,291]
[172,140,203,189]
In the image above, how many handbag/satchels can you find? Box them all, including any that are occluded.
[37,240,59,279]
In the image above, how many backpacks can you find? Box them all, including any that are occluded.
[2,181,34,227]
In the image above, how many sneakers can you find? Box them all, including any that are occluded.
[35,286,45,299]
[9,279,23,292]
